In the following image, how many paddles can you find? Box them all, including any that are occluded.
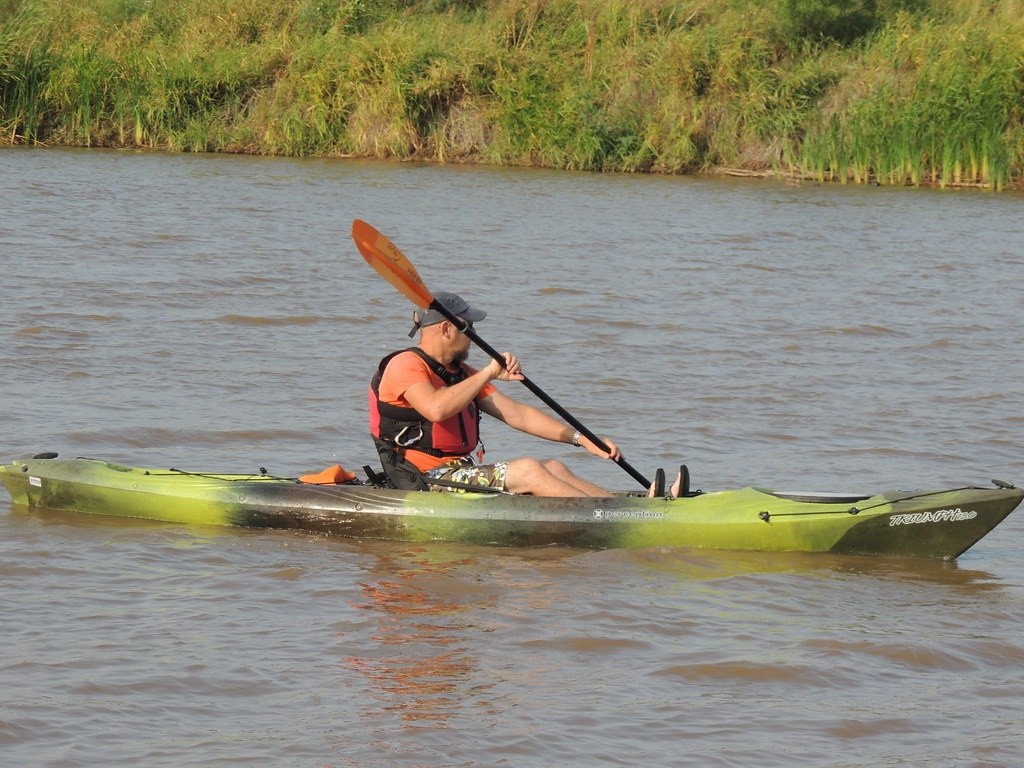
[349,216,653,491]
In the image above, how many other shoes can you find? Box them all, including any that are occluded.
[667,464,690,497]
[645,468,665,497]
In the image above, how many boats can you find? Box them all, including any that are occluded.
[0,448,1023,564]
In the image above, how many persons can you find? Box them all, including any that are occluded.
[370,290,691,498]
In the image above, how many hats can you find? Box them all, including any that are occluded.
[408,291,487,339]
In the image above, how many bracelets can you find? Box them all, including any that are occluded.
[572,429,581,448]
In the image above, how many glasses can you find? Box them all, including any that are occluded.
[439,320,473,330]
